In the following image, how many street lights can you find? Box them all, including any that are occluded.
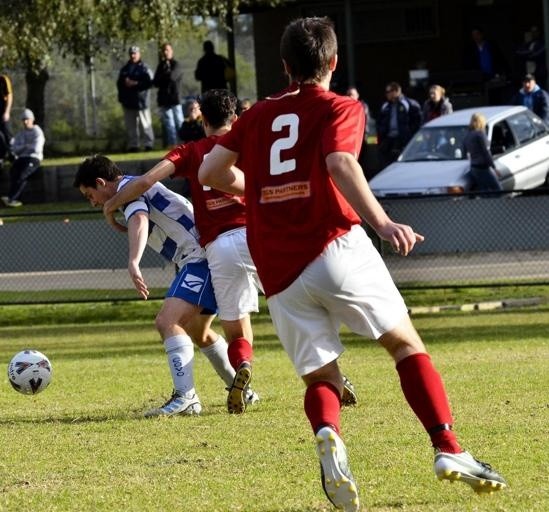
[82,0,100,140]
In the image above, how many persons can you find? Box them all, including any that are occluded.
[516,68,549,123]
[461,114,504,200]
[347,84,453,171]
[0,74,44,208]
[70,153,259,422]
[196,12,507,508]
[116,40,251,152]
[101,83,358,412]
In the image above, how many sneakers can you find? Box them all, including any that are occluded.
[2,197,22,207]
[225,361,259,414]
[435,447,508,497]
[341,374,358,406]
[145,388,202,418]
[315,427,359,512]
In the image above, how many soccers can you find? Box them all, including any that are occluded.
[8,349,52,394]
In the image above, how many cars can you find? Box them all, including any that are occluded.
[368,105,549,200]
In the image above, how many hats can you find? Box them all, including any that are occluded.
[20,109,35,120]
